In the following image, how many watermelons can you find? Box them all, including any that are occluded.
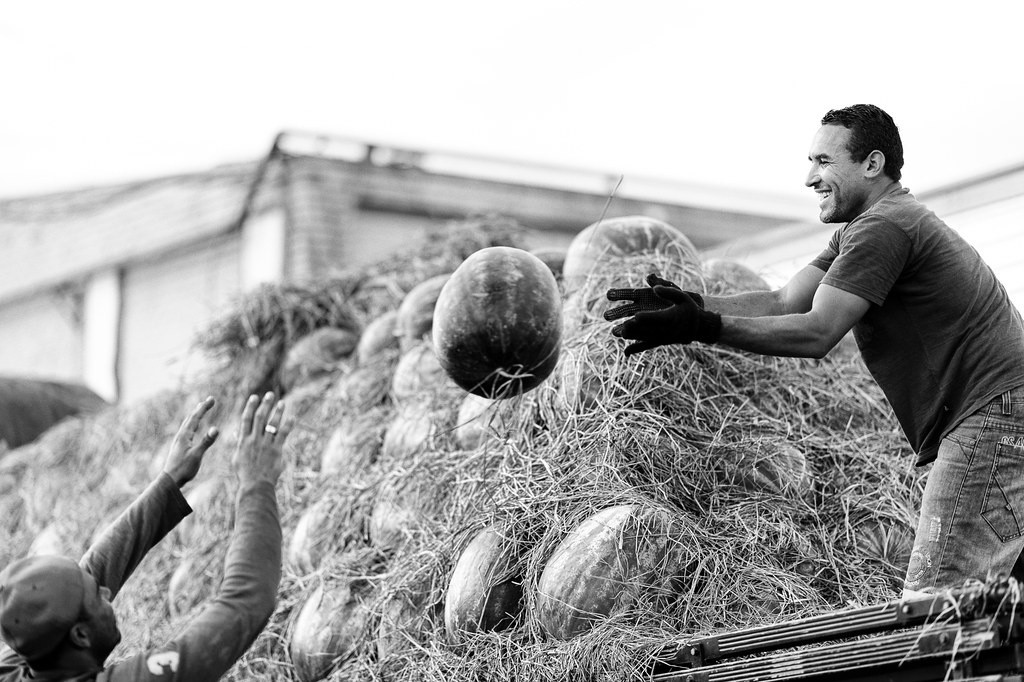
[273,215,917,682]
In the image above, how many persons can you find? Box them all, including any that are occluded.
[0,389,296,682]
[603,102,1024,605]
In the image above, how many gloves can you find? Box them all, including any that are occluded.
[621,286,721,358]
[602,274,703,337]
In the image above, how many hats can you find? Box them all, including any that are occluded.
[0,554,85,657]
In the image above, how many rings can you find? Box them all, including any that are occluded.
[265,424,277,434]
